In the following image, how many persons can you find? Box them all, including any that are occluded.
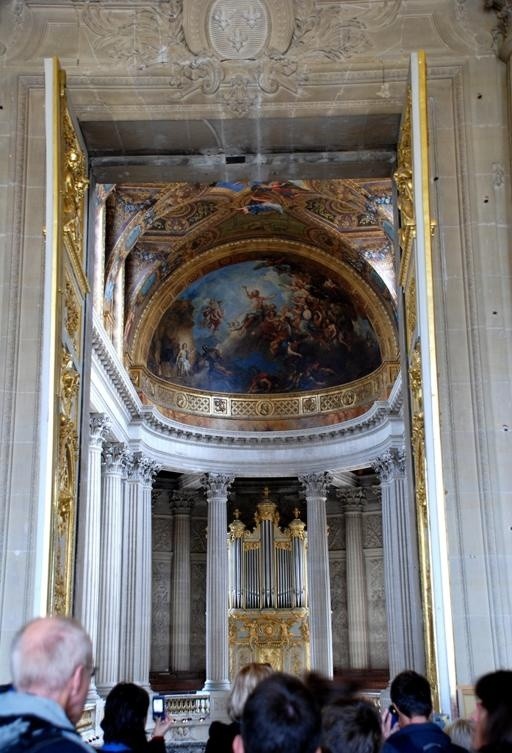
[174,273,360,390]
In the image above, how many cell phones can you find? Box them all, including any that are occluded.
[389,705,400,720]
[152,695,166,720]
